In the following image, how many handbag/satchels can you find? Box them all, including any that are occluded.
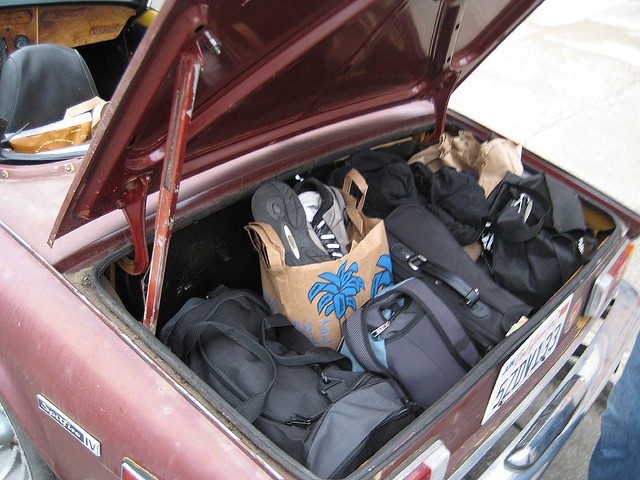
[160,282,418,478]
[243,168,395,351]
[384,202,535,357]
[487,170,590,308]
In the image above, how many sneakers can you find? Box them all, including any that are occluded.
[251,181,332,266]
[293,177,349,260]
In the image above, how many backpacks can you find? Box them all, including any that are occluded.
[335,277,484,414]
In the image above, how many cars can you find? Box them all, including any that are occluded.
[0,2,638,479]
